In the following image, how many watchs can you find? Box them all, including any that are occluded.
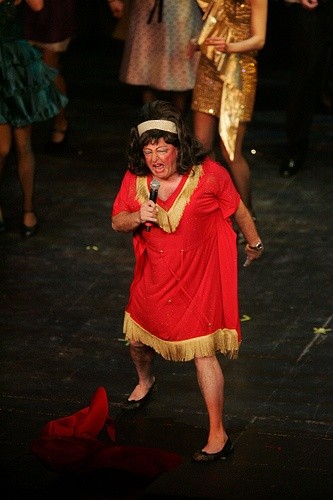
[247,241,263,252]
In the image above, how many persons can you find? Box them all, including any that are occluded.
[107,0,211,120]
[189,0,268,244]
[111,99,264,464]
[0,0,75,237]
[280,0,333,178]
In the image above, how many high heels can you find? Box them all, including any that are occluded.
[23,208,38,237]
[51,127,68,157]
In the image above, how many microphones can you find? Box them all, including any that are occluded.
[145,180,160,232]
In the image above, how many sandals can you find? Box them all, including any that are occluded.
[121,375,158,410]
[193,435,233,465]
